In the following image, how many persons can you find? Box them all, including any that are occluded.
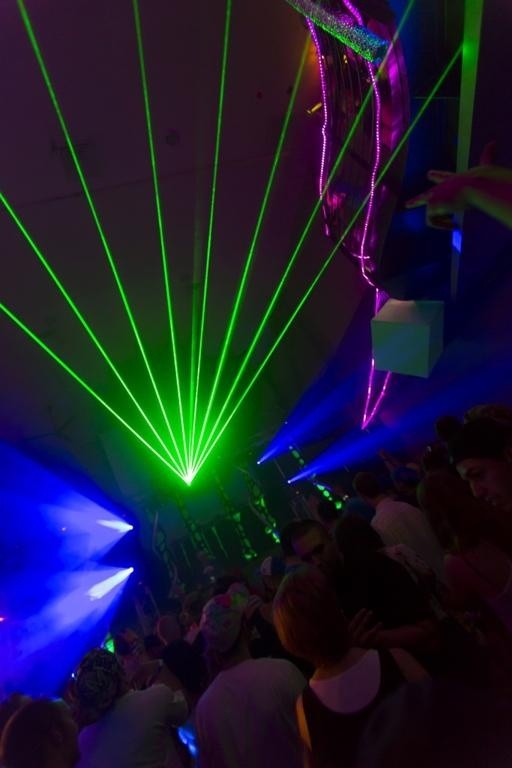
[0,405,512,768]
